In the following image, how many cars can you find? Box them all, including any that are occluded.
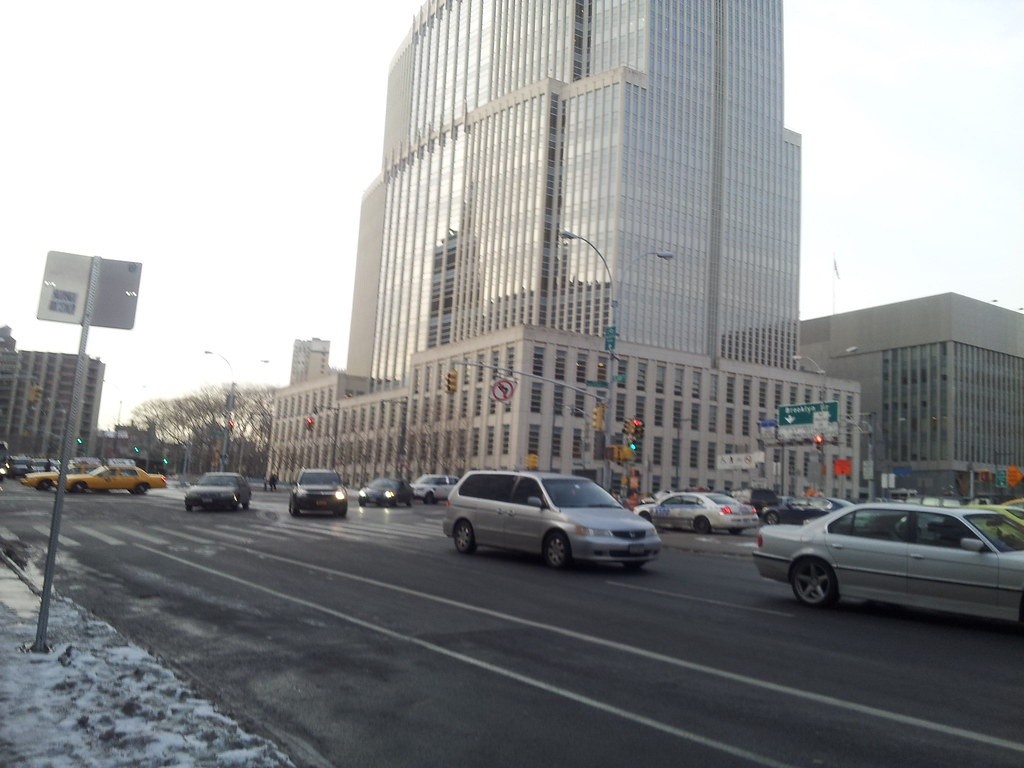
[633,487,872,534]
[961,499,1024,547]
[752,501,1024,627]
[19,466,167,495]
[184,472,459,512]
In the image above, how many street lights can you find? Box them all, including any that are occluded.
[206,350,269,469]
[790,348,860,494]
[558,229,675,488]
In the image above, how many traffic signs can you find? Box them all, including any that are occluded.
[777,401,839,433]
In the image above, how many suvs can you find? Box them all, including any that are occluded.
[286,466,348,517]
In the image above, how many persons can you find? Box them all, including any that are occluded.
[707,485,731,496]
[45,458,51,472]
[612,489,645,512]
[27,461,33,473]
[263,473,278,492]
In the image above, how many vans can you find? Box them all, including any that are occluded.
[0,441,134,481]
[441,471,662,570]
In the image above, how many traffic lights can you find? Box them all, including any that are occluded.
[29,385,44,404]
[525,454,539,468]
[591,405,605,430]
[229,419,234,430]
[446,371,457,392]
[306,417,313,430]
[604,414,642,487]
[815,433,824,452]
